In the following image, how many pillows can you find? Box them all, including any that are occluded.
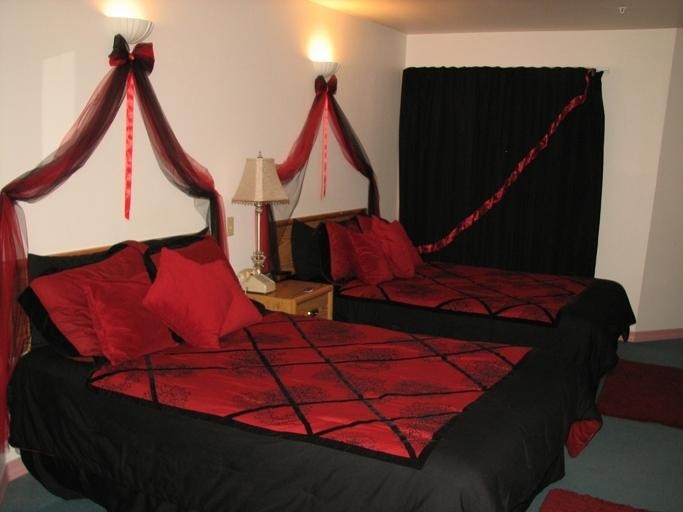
[371,220,423,278]
[31,247,151,356]
[152,237,262,337]
[201,260,261,340]
[327,222,360,280]
[80,272,178,368]
[350,235,393,282]
[358,216,388,231]
[142,247,232,351]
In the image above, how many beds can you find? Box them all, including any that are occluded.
[277,207,636,423]
[7,230,565,510]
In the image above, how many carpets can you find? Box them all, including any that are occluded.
[598,359,683,429]
[537,488,650,512]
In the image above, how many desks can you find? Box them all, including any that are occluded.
[245,280,333,323]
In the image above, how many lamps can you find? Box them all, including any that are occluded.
[233,157,288,270]
[312,61,339,79]
[110,14,154,44]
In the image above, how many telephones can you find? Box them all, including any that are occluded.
[237,269,276,294]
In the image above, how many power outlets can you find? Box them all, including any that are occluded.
[226,217,233,235]
